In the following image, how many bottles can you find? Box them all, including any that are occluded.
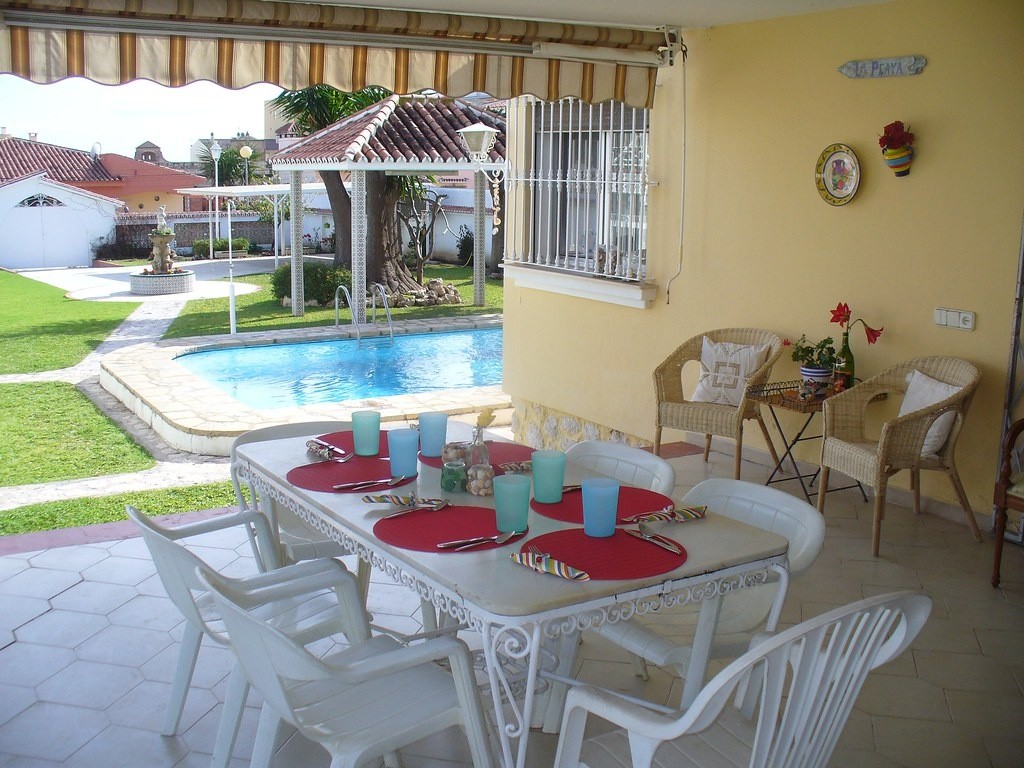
[440,462,467,493]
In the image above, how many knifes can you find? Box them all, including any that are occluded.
[312,437,346,454]
[624,528,681,554]
[436,533,523,548]
[333,479,391,489]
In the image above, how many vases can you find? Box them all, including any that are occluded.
[799,364,834,398]
[882,146,913,177]
[467,426,494,496]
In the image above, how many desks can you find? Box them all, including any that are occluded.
[746,377,868,505]
[230,420,791,768]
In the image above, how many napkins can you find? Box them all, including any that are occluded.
[306,440,334,461]
[634,505,708,521]
[490,460,531,476]
[360,490,455,512]
[508,552,591,582]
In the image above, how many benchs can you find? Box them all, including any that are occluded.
[214,250,248,258]
[286,247,315,255]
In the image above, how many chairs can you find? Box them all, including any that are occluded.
[990,418,1024,588]
[817,355,984,556]
[652,327,783,480]
[125,421,933,768]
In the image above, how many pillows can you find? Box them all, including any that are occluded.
[690,335,770,406]
[899,368,964,454]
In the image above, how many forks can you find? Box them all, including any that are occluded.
[384,497,449,519]
[333,452,354,462]
[616,510,662,521]
[529,544,541,554]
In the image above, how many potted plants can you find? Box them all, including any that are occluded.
[146,228,176,244]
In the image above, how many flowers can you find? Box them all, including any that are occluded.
[830,302,884,345]
[466,407,497,453]
[783,333,836,369]
[879,119,915,149]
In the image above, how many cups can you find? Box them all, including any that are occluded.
[419,412,449,457]
[531,450,566,503]
[387,428,418,478]
[493,474,531,533]
[582,478,619,537]
[352,411,380,456]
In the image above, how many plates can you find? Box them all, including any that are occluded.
[815,143,860,206]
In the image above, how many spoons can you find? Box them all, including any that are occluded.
[455,531,516,551]
[639,523,678,548]
[352,475,405,490]
